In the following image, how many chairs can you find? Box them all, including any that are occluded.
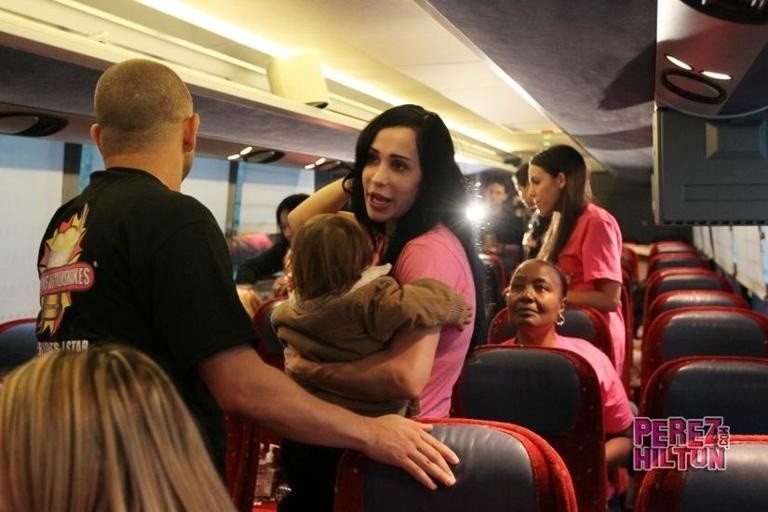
[0,313,39,386]
[224,232,767,512]
